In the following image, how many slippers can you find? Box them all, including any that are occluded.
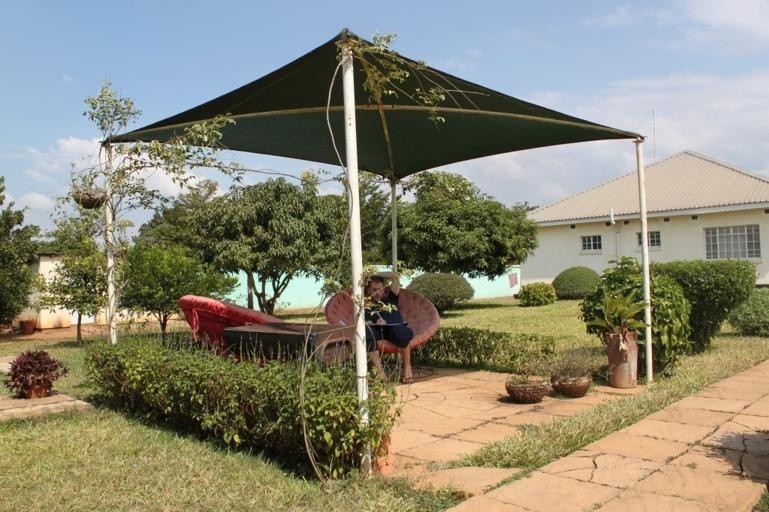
[402,375,414,384]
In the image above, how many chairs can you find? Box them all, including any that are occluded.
[178,294,281,362]
[325,287,440,382]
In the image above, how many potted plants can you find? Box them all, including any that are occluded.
[3,351,69,399]
[505,286,651,404]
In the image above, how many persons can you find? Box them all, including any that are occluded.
[363,268,414,383]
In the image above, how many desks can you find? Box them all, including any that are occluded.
[223,322,356,373]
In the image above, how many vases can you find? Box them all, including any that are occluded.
[20,319,35,334]
